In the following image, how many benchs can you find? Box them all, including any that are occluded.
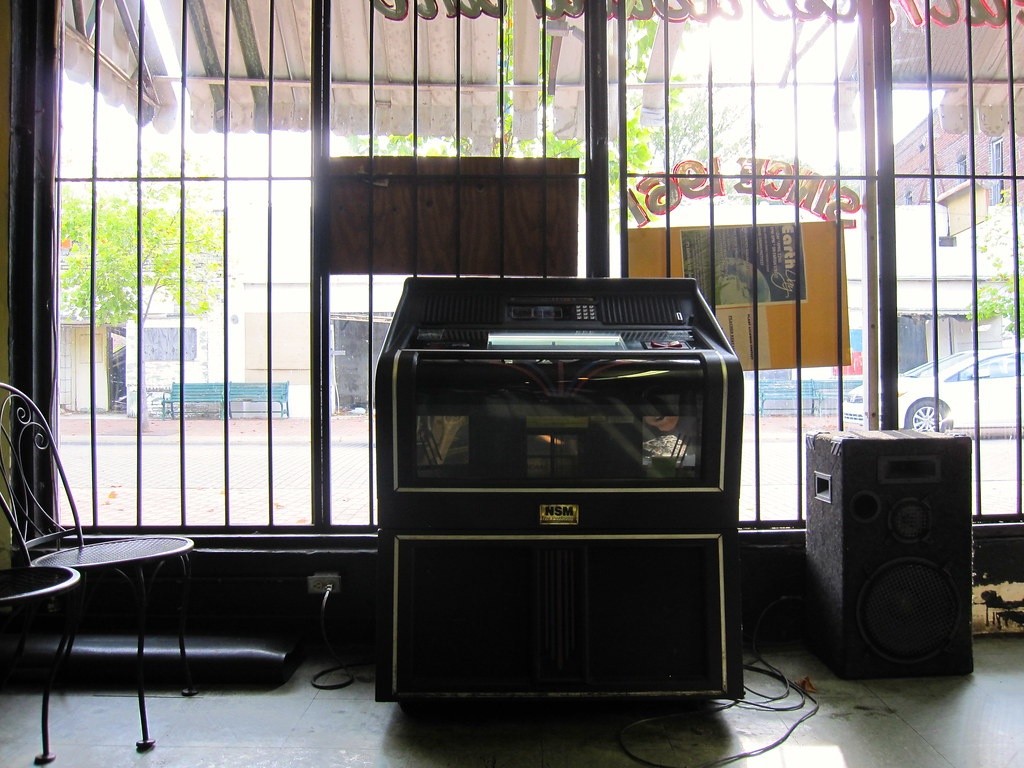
[758,378,862,417]
[162,382,289,419]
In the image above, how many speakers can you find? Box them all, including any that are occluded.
[804,432,973,679]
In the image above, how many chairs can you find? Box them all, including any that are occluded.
[0,566,84,763]
[0,383,195,749]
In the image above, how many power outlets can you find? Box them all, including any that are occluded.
[307,571,342,595]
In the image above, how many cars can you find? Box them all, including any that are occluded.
[843,349,1024,440]
